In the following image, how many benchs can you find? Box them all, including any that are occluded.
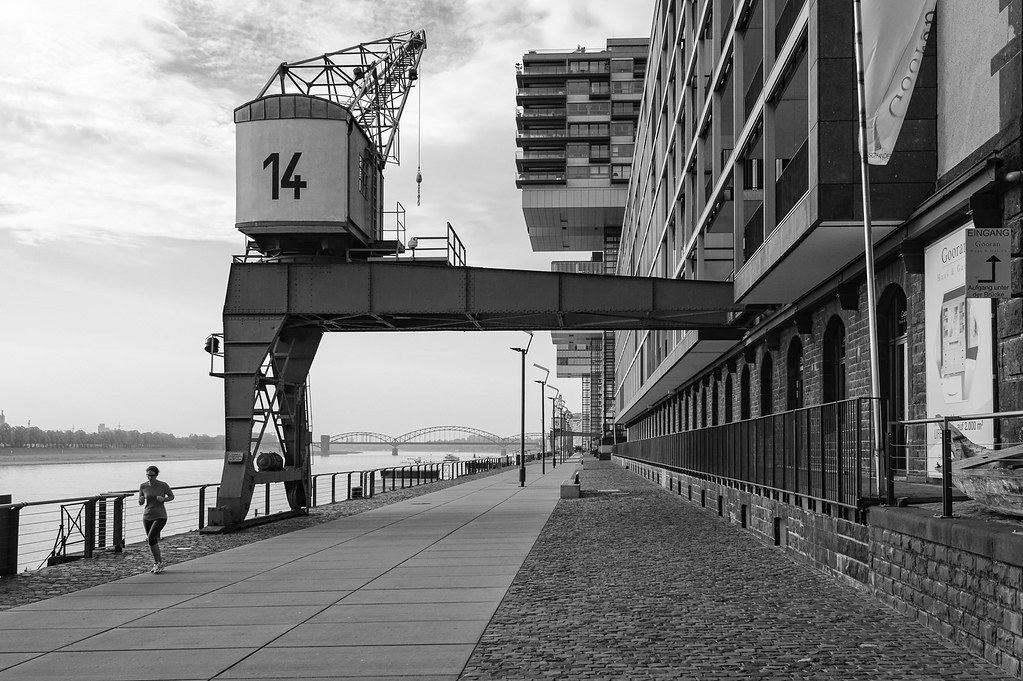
[560,471,580,499]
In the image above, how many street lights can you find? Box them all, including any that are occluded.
[546,385,559,467]
[533,364,550,474]
[555,395,574,463]
[509,331,533,487]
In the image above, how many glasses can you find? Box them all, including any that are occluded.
[146,474,156,477]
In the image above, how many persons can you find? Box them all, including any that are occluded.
[138,466,174,573]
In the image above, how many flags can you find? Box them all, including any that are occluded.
[860,0,938,165]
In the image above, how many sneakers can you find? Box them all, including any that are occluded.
[151,561,164,574]
